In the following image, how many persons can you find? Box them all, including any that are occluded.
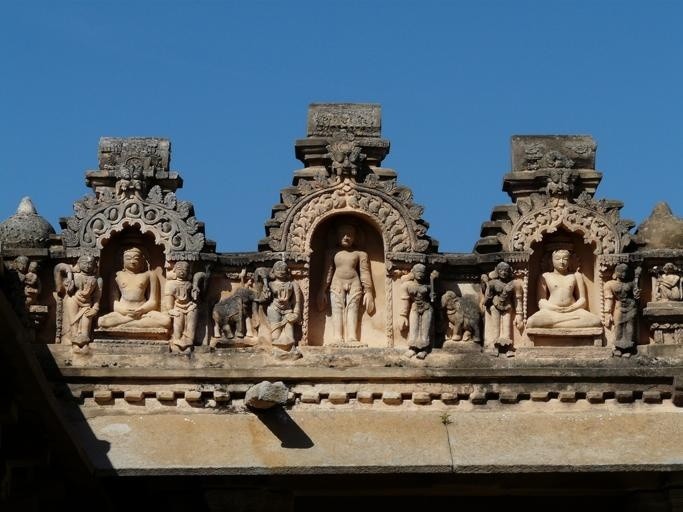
[480,261,523,345]
[525,247,601,328]
[654,262,682,300]
[96,246,172,328]
[264,261,303,346]
[602,263,642,358]
[13,254,42,307]
[397,263,436,361]
[316,218,376,342]
[68,256,103,353]
[21,261,39,304]
[167,260,199,350]
